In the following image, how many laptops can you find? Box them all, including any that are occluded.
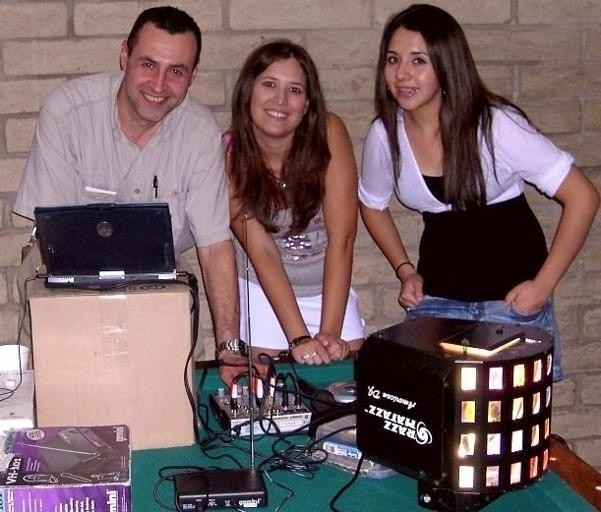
[34,203,177,287]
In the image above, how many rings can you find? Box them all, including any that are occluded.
[302,352,311,361]
[311,349,319,358]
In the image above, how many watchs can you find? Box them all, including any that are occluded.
[215,336,250,363]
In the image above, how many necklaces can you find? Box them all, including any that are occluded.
[267,159,290,190]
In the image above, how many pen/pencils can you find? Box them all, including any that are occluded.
[152,175,158,199]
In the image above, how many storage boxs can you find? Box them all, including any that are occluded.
[1,420,134,512]
[28,267,203,459]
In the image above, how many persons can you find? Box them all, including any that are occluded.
[10,6,260,390]
[359,5,601,387]
[222,38,370,365]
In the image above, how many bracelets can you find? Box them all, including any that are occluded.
[396,259,416,278]
[287,333,313,351]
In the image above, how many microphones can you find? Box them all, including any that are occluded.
[297,378,335,412]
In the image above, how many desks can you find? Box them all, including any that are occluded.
[0,341,600,512]
